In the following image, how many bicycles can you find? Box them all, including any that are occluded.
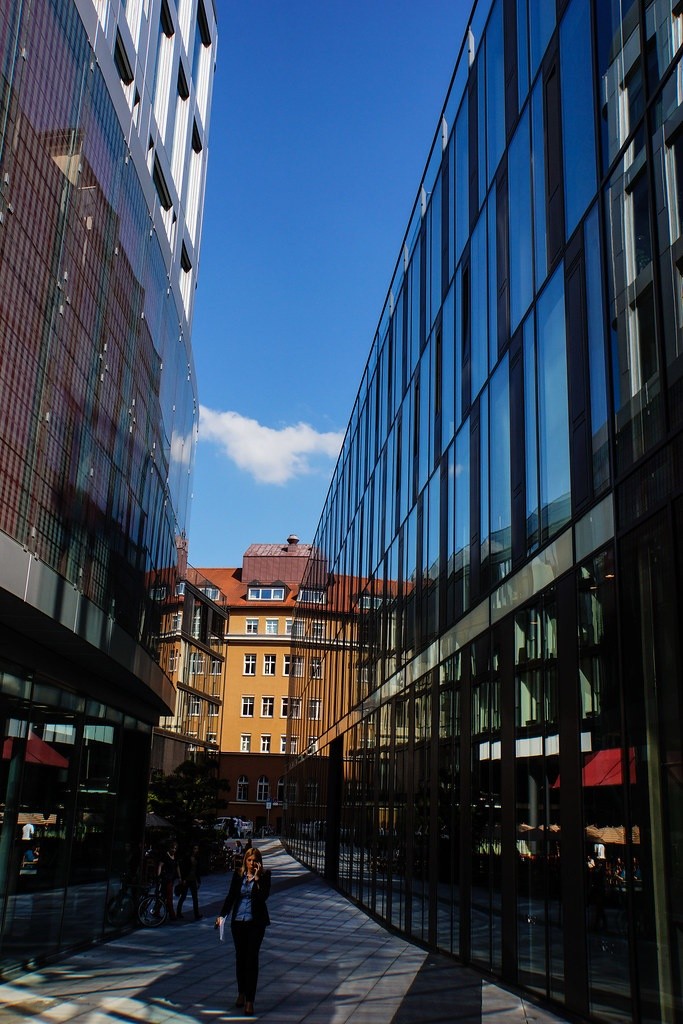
[138,871,169,928]
[107,872,138,929]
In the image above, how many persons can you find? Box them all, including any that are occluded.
[19,824,40,868]
[215,847,271,1016]
[150,841,182,923]
[176,845,203,921]
[614,864,643,882]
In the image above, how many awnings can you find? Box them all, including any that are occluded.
[1,728,70,768]
[552,744,683,790]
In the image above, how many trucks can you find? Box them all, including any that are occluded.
[214,817,253,838]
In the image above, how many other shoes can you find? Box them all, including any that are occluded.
[194,914,204,920]
[235,993,245,1007]
[245,1001,253,1015]
[177,912,184,919]
